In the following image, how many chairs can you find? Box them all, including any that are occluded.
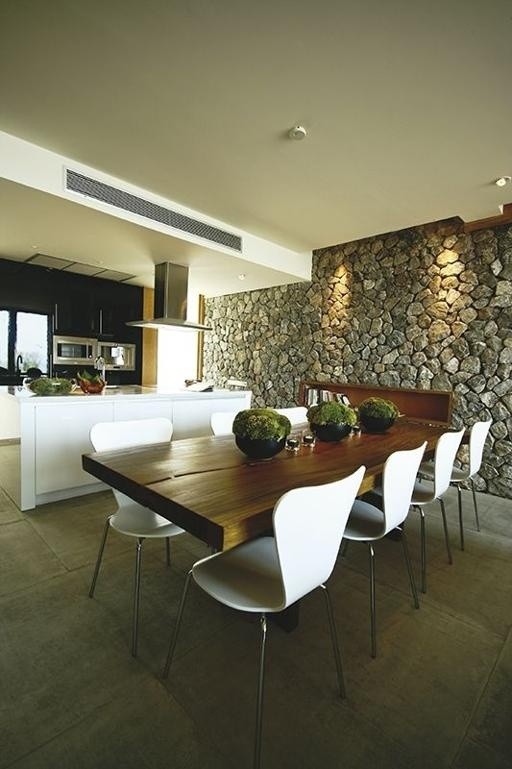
[370,422,467,594]
[161,463,368,767]
[86,414,216,660]
[413,417,495,552]
[270,402,310,429]
[338,435,434,666]
[209,406,249,437]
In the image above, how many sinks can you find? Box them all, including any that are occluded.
[105,384,120,391]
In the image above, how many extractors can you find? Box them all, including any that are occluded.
[123,261,214,333]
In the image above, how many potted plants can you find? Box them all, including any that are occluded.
[304,402,359,446]
[356,391,399,436]
[230,406,292,464]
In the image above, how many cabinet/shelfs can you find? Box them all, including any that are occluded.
[96,277,139,342]
[0,385,22,444]
[50,269,96,338]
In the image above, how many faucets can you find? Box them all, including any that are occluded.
[16,354,23,375]
[92,354,108,387]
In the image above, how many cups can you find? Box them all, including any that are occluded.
[351,425,362,437]
[284,429,318,452]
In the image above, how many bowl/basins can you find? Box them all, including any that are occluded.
[234,433,287,461]
[309,422,351,441]
[29,381,77,395]
[78,381,105,393]
[360,413,395,433]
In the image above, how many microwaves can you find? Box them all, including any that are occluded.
[94,341,138,372]
[52,335,98,367]
